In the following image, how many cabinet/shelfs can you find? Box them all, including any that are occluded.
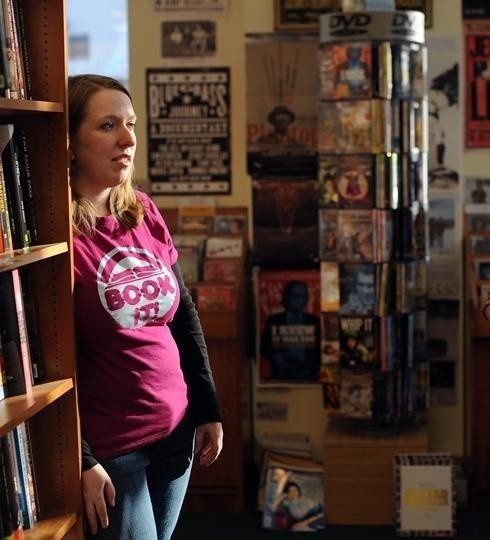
[323,422,428,526]
[155,206,247,511]
[0,1,90,538]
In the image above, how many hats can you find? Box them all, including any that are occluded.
[268,106,294,125]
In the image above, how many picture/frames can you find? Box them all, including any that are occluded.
[146,66,232,196]
[274,0,430,30]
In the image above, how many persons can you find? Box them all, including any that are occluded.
[68,73,225,540]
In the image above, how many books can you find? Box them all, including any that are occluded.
[0,0,47,540]
[244,42,456,538]
[172,211,247,314]
